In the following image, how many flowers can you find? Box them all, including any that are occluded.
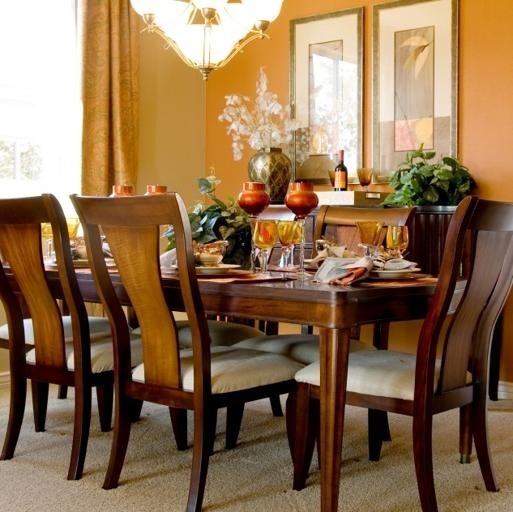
[217,64,303,161]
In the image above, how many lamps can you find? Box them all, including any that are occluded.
[130,0,282,82]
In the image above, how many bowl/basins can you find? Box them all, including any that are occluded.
[198,240,229,267]
[407,262,418,269]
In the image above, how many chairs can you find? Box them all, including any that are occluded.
[71,194,319,512]
[313,206,418,267]
[294,196,513,512]
[0,193,143,482]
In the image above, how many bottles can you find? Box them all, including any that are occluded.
[333,150,347,192]
[142,185,168,257]
[107,185,133,198]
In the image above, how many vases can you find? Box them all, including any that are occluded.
[247,148,291,205]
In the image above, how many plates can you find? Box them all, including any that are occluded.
[385,259,411,270]
[196,265,242,276]
[373,270,420,278]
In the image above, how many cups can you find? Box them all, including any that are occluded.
[65,218,79,240]
[356,221,383,247]
[357,167,373,191]
[387,226,408,258]
[277,221,298,267]
[40,222,54,261]
[252,219,277,275]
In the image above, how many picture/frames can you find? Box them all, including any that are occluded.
[288,6,364,185]
[371,0,458,185]
